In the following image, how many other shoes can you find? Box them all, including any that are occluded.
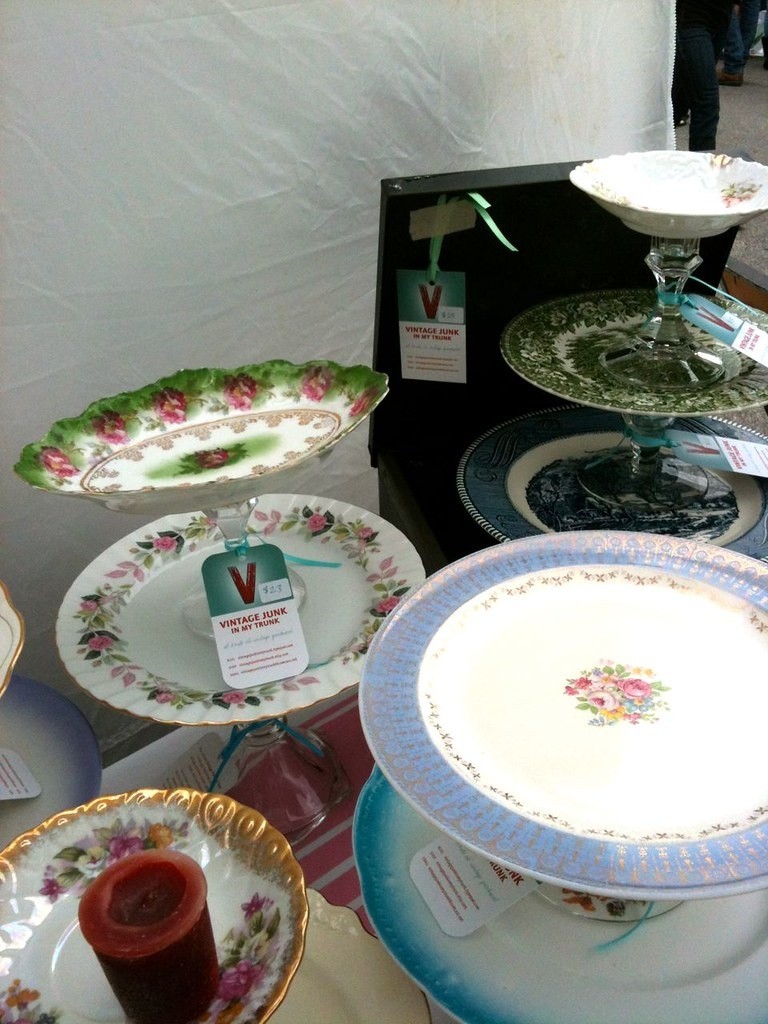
[673,109,689,125]
[716,69,744,87]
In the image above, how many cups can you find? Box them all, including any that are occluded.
[77,852,219,1024]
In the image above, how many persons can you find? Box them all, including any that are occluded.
[716,0,760,87]
[671,0,737,151]
[762,0,768,71]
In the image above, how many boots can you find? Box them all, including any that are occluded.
[762,36,768,70]
